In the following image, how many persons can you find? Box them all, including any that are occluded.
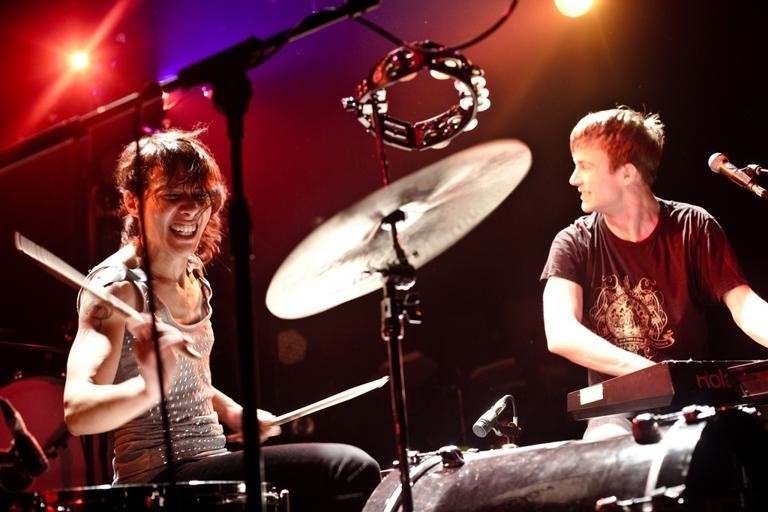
[63,127,382,512]
[538,104,768,440]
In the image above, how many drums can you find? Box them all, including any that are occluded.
[59,480,246,512]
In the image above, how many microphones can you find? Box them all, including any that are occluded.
[708,152,767,198]
[472,398,506,438]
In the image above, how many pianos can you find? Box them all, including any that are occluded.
[565,358,768,420]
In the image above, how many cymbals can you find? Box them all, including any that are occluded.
[266,140,530,320]
